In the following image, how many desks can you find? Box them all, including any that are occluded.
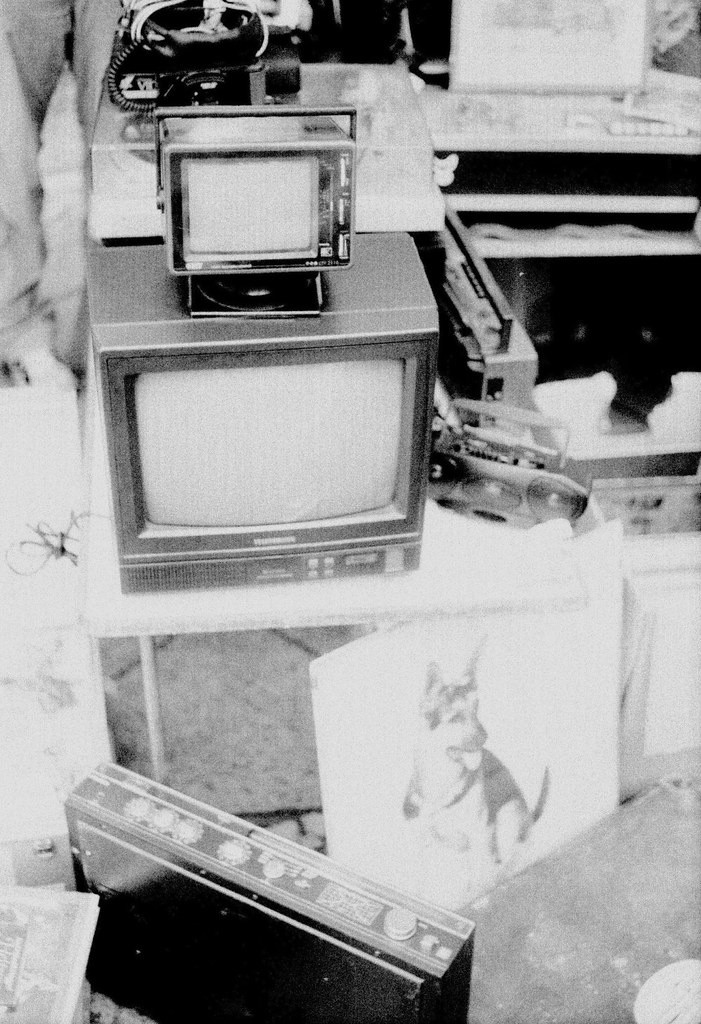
[70,497,516,791]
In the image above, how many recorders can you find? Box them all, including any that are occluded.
[428,397,592,528]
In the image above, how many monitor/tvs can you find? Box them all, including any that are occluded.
[153,103,361,318]
[82,230,441,595]
